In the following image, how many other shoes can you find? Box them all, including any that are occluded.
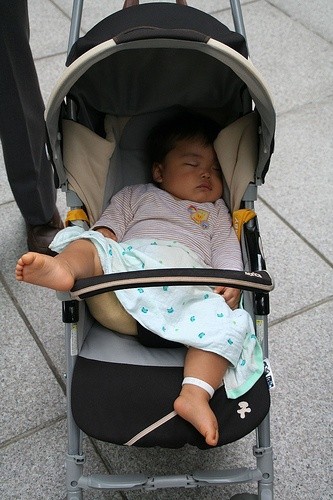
[23,206,63,257]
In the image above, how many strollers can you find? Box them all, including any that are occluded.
[43,0,276,500]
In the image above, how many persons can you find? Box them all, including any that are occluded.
[0,0,65,257]
[14,120,243,446]
